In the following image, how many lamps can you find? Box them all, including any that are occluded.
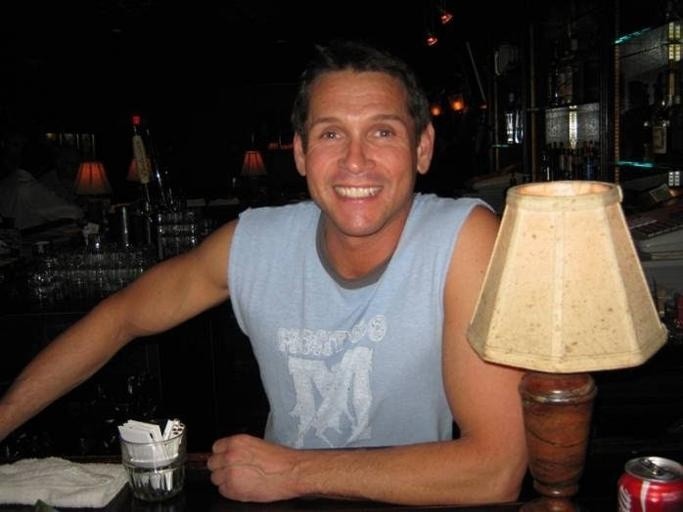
[71,160,114,228]
[466,180,671,512]
[241,150,270,201]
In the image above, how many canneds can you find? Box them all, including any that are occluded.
[617,456,683,512]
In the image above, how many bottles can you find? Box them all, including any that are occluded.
[543,140,600,179]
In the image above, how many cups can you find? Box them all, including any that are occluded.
[118,422,186,501]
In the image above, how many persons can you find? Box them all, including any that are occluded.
[0,42,533,507]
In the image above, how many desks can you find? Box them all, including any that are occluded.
[0,446,680,510]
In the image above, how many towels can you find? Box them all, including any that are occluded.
[0,456,130,510]
